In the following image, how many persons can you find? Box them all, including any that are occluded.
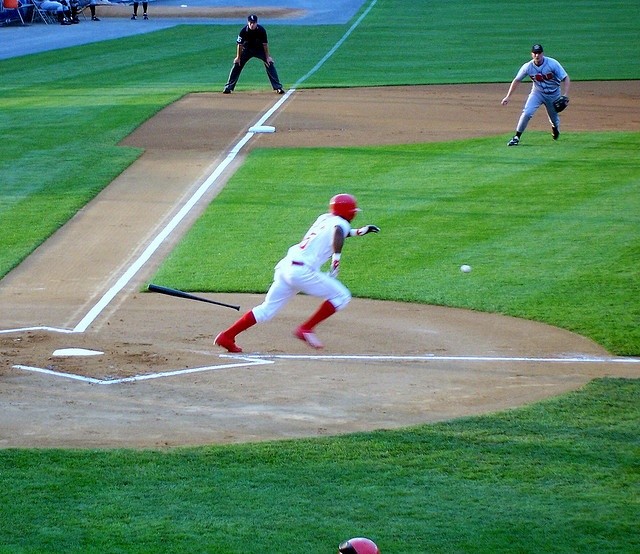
[213,192,381,352]
[222,13,285,95]
[131,0,150,21]
[70,0,101,24]
[501,43,571,147]
[33,0,79,25]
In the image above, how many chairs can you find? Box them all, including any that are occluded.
[29,0,89,23]
[1,0,34,24]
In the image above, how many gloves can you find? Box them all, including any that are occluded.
[330,253,341,277]
[358,226,381,236]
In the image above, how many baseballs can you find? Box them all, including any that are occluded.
[461,265,471,273]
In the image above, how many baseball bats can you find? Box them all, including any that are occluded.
[148,284,240,311]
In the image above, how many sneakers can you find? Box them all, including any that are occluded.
[224,90,231,94]
[508,137,520,147]
[214,332,243,353]
[552,125,559,139]
[295,328,326,349]
[278,89,284,95]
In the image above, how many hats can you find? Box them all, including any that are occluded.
[533,45,544,54]
[248,15,258,23]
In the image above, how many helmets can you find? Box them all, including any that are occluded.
[329,195,357,221]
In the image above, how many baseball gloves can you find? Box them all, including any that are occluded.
[553,96,570,113]
